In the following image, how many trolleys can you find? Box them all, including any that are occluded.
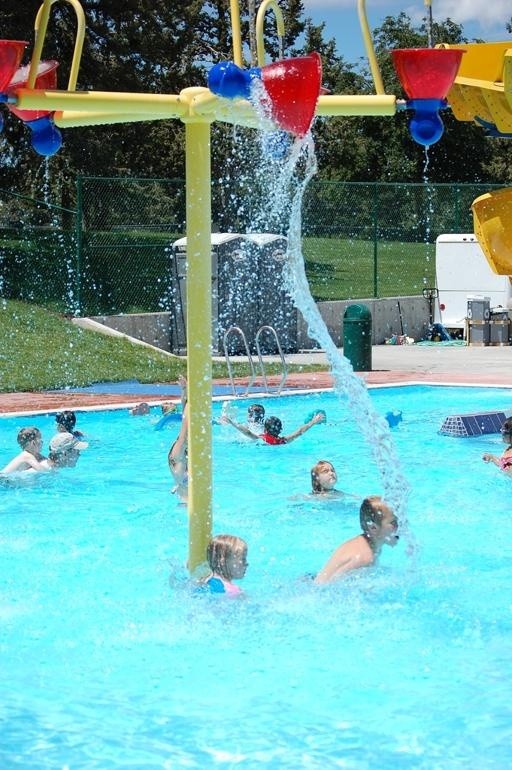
[421,287,443,342]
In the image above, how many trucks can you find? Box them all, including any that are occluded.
[433,232,512,328]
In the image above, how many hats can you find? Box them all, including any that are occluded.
[49,433,88,453]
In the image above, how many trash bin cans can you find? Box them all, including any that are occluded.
[343,304,373,372]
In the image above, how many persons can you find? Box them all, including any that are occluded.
[30,432,89,471]
[190,534,248,601]
[167,373,189,508]
[156,401,182,428]
[129,402,150,416]
[1,426,47,471]
[222,411,326,448]
[294,460,349,501]
[313,496,399,583]
[481,416,511,478]
[55,410,85,441]
[241,404,266,426]
